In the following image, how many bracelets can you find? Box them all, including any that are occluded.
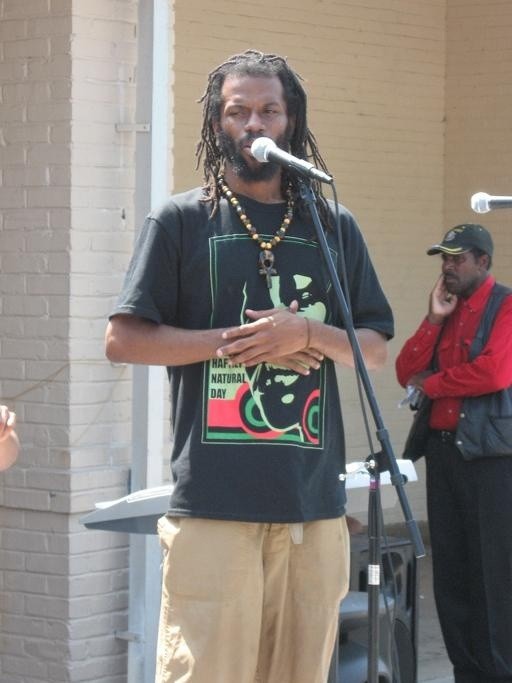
[304,317,312,350]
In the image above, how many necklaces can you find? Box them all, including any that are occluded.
[218,177,297,290]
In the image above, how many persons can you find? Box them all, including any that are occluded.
[393,224,512,682]
[0,403,21,473]
[103,52,395,683]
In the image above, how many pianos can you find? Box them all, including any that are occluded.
[77,458,417,534]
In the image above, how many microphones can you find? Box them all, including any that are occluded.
[248,137,334,185]
[469,191,512,214]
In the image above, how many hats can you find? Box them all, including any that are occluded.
[427,224,493,258]
